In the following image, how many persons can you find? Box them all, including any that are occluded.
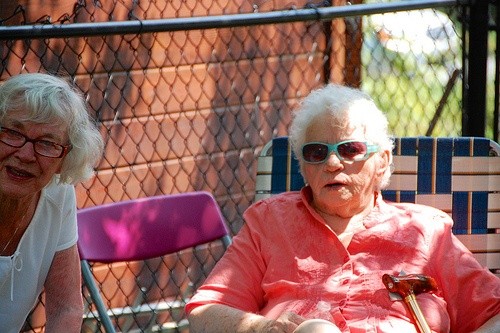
[1,73,106,333]
[182,86,500,333]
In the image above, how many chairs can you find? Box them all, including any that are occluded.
[76,191,233,332]
[255,135,500,279]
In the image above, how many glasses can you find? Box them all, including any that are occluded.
[0,126,74,159]
[300,139,379,164]
